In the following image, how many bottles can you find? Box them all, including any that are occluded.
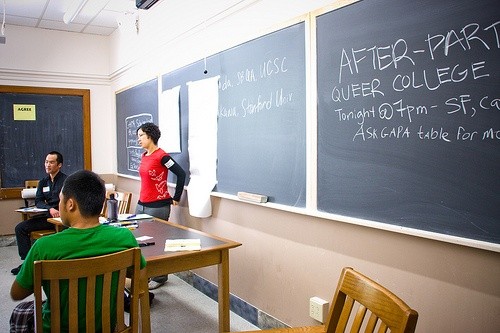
[107,193,118,222]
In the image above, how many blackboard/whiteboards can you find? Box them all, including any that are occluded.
[115,0,500,252]
[0,85,92,199]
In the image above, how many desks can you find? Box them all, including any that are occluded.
[110,217,242,333]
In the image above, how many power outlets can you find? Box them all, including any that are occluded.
[309,296,329,323]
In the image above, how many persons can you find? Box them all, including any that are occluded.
[11,151,68,275]
[136,122,186,289]
[9,170,147,333]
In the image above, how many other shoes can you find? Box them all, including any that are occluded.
[11,264,22,274]
[147,280,163,289]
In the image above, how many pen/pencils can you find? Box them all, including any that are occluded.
[139,242,155,247]
[127,214,136,219]
[129,227,136,230]
[121,223,137,226]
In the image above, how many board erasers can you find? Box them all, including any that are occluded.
[238,192,268,203]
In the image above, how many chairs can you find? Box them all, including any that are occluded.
[21,180,131,240]
[34,246,141,333]
[224,266,418,333]
